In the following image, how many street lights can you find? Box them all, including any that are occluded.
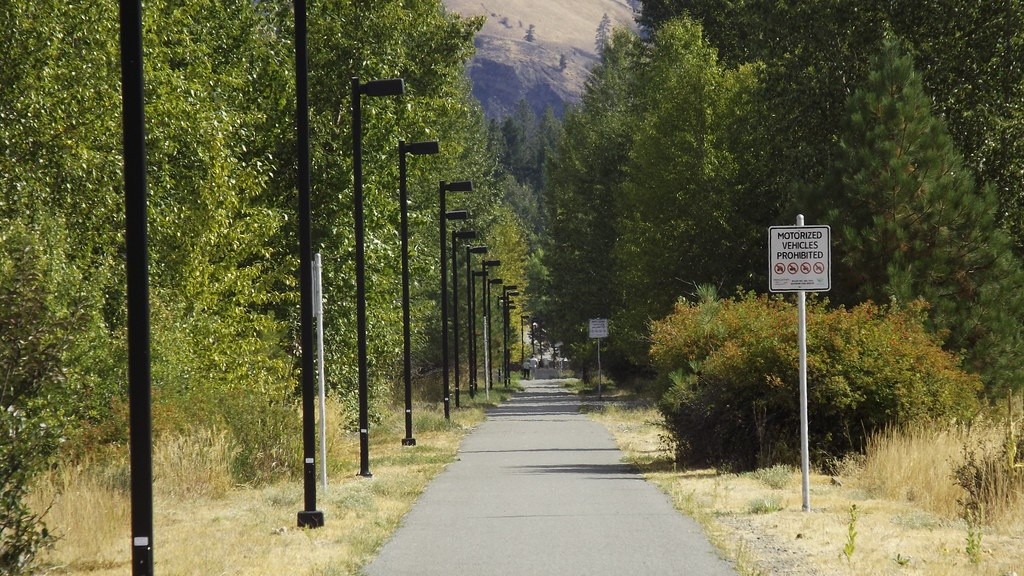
[471,268,490,393]
[482,259,519,390]
[350,77,406,476]
[451,229,477,408]
[438,179,473,421]
[531,319,539,355]
[398,137,440,445]
[521,314,530,365]
[466,246,488,399]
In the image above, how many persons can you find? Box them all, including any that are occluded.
[522,354,539,380]
